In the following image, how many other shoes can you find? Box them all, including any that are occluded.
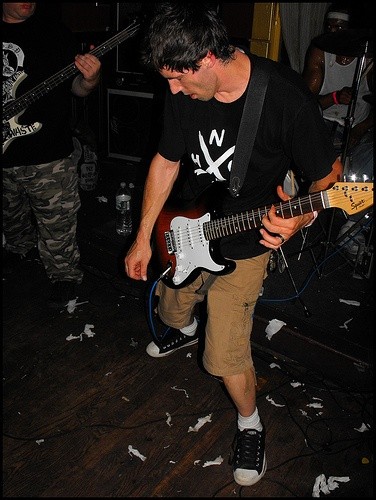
[47,279,76,308]
[0,246,37,264]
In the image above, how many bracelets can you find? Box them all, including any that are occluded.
[332,92,339,104]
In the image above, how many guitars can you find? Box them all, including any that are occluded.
[148,172,373,290]
[2,15,146,151]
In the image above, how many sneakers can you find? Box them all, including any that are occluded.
[145,320,202,359]
[229,420,268,487]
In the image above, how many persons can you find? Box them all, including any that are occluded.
[1,2,105,327]
[122,3,375,487]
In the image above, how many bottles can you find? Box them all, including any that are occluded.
[116,180,132,236]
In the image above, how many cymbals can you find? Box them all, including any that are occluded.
[313,29,374,58]
